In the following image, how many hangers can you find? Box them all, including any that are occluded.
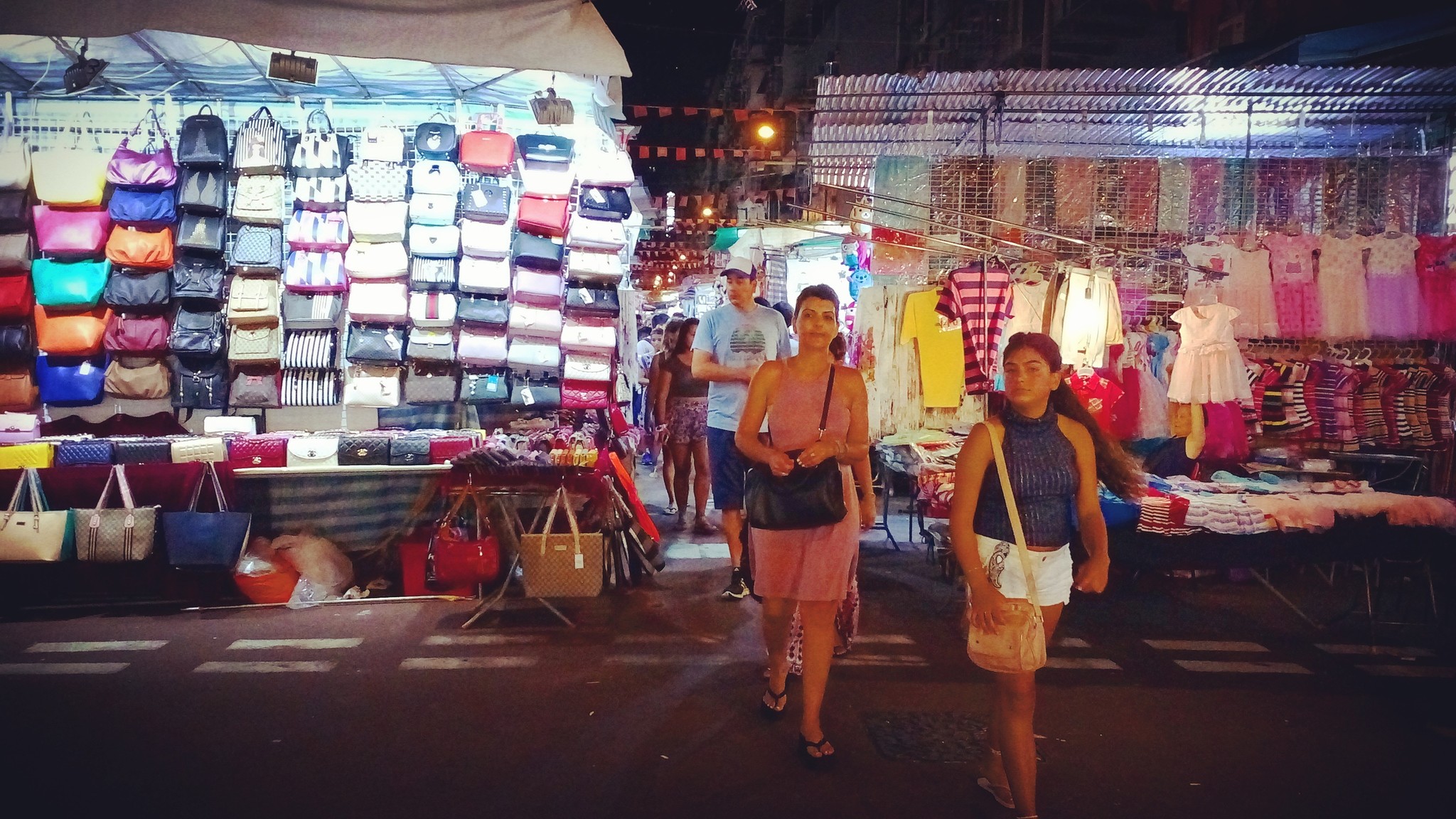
[935,271,949,288]
[1329,215,1357,239]
[975,244,1042,284]
[1238,231,1273,254]
[1379,214,1405,237]
[1052,251,1113,279]
[1241,343,1441,367]
[1121,313,1167,332]
[1196,223,1223,244]
[1277,214,1304,236]
[1185,272,1217,310]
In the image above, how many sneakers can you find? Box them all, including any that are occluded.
[641,452,653,465]
[721,567,750,599]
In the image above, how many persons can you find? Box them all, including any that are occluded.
[735,286,870,766]
[949,332,1146,819]
[637,313,687,514]
[763,333,877,681]
[657,318,719,533]
[690,256,792,598]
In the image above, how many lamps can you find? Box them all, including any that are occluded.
[265,50,320,86]
[64,40,110,94]
[527,72,576,125]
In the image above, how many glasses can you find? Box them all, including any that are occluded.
[652,338,661,341]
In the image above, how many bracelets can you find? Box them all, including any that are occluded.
[835,440,849,459]
[866,492,876,499]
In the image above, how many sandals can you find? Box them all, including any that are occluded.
[674,513,687,531]
[694,521,719,534]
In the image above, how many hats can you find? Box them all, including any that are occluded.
[720,257,756,278]
[674,313,687,318]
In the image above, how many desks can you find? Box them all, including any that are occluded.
[872,458,1456,660]
[447,488,607,630]
[0,461,452,592]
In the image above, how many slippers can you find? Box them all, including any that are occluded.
[761,687,787,718]
[663,505,677,514]
[978,777,1015,809]
[799,732,834,762]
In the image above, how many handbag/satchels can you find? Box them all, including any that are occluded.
[0,411,605,475]
[967,599,1046,673]
[0,452,665,596]
[0,97,632,410]
[745,449,848,528]
